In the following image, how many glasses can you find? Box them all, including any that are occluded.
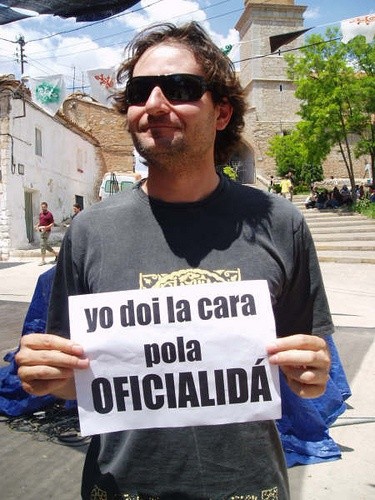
[124,73,225,106]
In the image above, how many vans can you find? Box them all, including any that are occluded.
[98,172,141,202]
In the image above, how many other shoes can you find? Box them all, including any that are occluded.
[54,256,58,263]
[38,262,46,266]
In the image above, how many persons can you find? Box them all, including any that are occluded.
[279,171,295,202]
[71,203,81,220]
[270,175,275,193]
[304,185,375,209]
[15,20,336,500]
[37,202,59,266]
